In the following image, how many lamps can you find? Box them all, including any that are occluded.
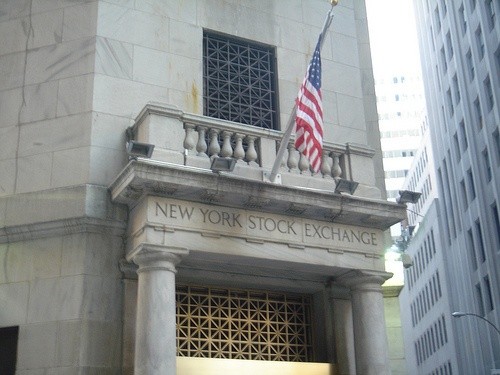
[209,156,237,174]
[334,178,360,196]
[126,140,155,159]
[397,190,422,205]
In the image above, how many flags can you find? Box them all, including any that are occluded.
[294,30,324,174]
